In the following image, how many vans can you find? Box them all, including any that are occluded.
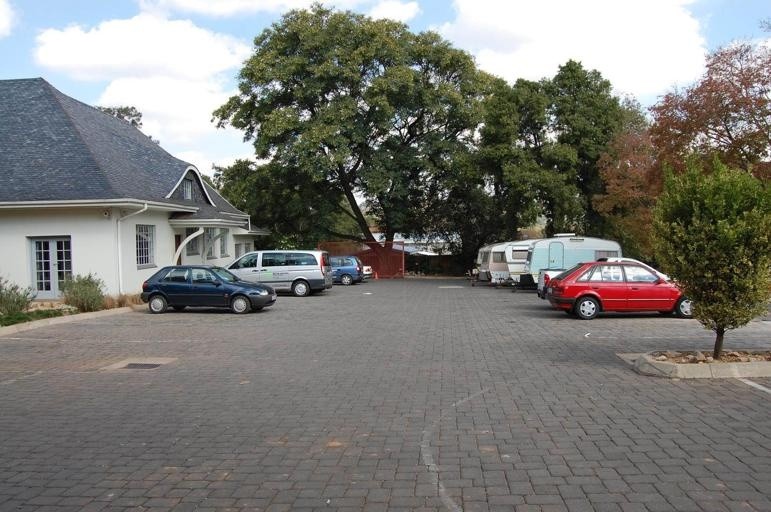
[226,249,373,296]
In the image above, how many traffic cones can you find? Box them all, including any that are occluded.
[374,272,378,280]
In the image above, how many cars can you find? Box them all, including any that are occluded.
[140,265,277,314]
[545,261,700,321]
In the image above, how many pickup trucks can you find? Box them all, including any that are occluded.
[536,257,672,302]
